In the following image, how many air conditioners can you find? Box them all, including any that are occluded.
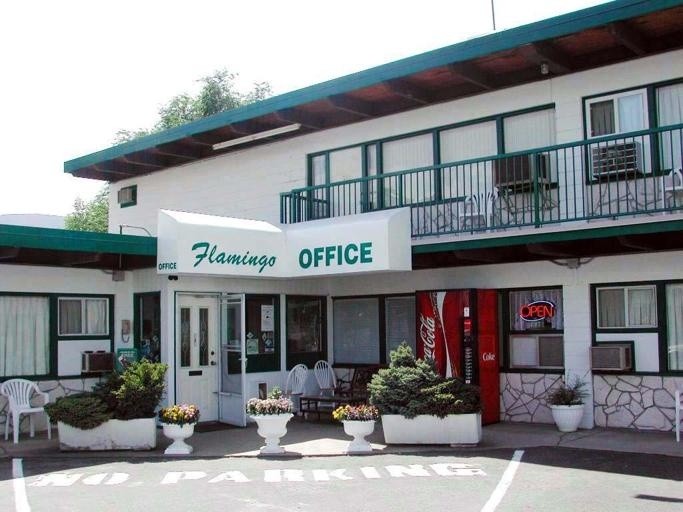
[592,141,642,178]
[494,154,549,188]
[590,343,633,371]
[82,352,115,373]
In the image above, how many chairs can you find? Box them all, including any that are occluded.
[661,167,683,215]
[283,359,372,421]
[458,184,499,232]
[0,378,52,444]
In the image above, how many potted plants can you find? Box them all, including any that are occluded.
[43,357,169,452]
[547,376,592,433]
[366,340,483,448]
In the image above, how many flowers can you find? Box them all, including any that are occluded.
[159,403,201,428]
[246,398,294,417]
[332,404,379,421]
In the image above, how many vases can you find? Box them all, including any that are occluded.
[249,413,295,454]
[161,422,197,455]
[341,420,376,452]
